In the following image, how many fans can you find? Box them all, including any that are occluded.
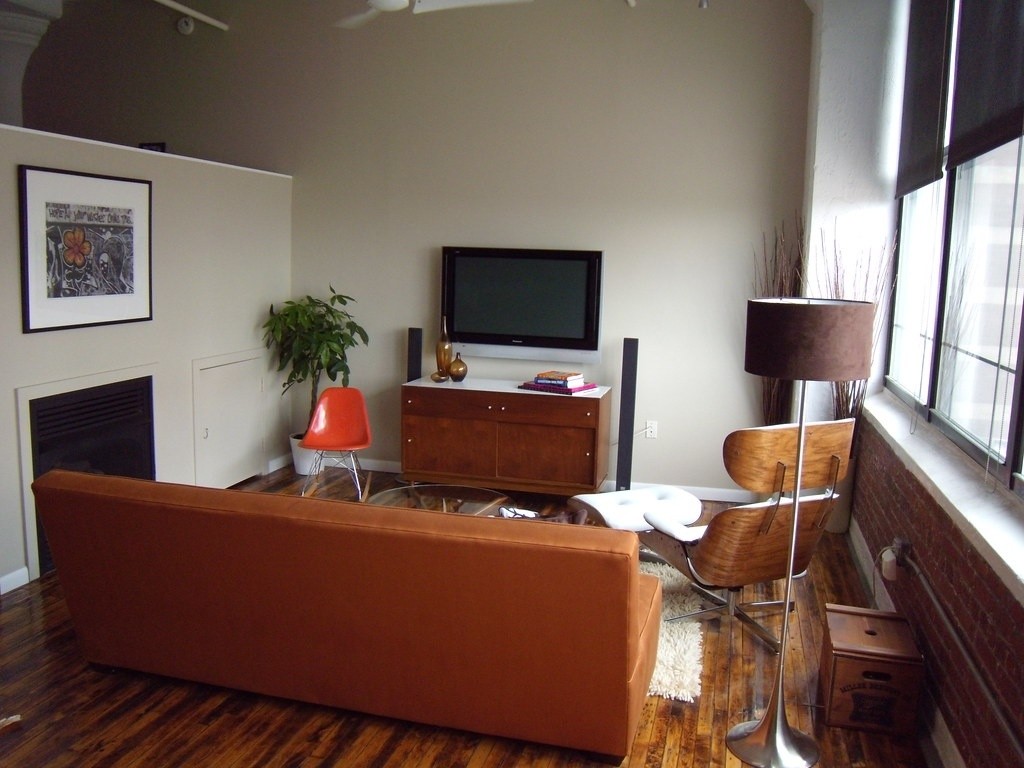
[330,0,516,30]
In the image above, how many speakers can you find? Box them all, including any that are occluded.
[407,328,422,381]
[616,337,640,491]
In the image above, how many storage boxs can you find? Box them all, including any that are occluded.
[819,602,924,737]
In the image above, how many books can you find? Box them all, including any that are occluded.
[537,371,583,380]
[518,377,598,395]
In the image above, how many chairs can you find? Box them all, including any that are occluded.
[298,387,372,502]
[643,418,856,655]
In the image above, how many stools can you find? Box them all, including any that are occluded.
[569,484,703,532]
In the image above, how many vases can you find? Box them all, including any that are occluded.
[436,316,452,376]
[449,352,467,381]
[821,460,861,534]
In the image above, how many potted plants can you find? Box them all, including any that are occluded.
[261,285,369,476]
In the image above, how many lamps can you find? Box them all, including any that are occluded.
[725,297,873,768]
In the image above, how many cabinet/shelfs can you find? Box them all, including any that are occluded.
[402,372,612,497]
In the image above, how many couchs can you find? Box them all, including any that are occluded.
[31,468,663,767]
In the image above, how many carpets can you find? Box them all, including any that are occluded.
[638,561,706,704]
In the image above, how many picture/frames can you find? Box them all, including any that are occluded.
[17,164,153,334]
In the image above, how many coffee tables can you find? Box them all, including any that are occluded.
[365,485,517,519]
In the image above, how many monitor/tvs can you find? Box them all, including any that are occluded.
[441,247,603,364]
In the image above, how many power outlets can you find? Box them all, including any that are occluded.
[891,537,912,565]
[646,421,657,438]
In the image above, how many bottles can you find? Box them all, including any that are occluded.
[448,352,468,382]
[436,316,452,377]
